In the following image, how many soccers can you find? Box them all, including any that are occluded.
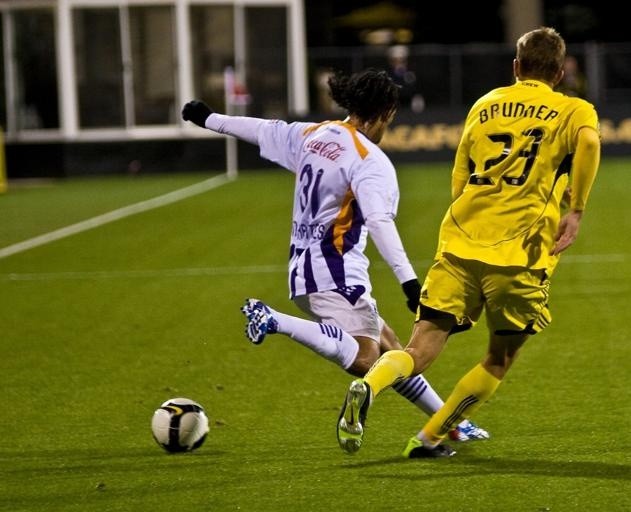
[152,398,210,454]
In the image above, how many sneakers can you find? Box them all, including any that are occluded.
[448,421,491,442]
[239,297,277,345]
[401,435,459,459]
[336,377,373,454]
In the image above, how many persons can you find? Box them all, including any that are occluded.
[552,42,589,96]
[335,27,601,459]
[182,67,490,443]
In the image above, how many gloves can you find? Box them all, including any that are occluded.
[401,278,423,314]
[182,100,214,129]
